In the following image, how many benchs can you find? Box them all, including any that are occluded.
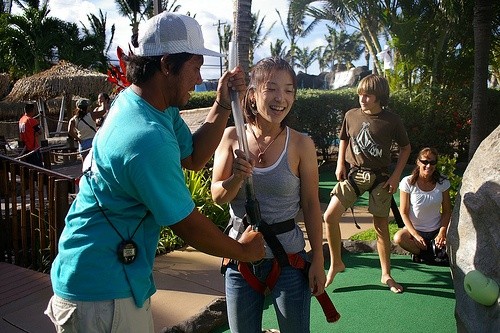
[5,135,70,161]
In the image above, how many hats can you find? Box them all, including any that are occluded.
[76,98,89,108]
[129,10,227,57]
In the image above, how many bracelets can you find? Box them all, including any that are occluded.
[215,100,232,111]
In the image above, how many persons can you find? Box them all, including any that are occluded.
[18,103,49,194]
[68,98,110,163]
[323,74,404,294]
[376,45,394,72]
[210,56,327,333]
[393,146,453,263]
[43,11,267,333]
[92,92,113,131]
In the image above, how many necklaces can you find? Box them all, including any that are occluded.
[248,123,285,163]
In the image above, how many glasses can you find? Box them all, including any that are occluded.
[419,159,437,165]
